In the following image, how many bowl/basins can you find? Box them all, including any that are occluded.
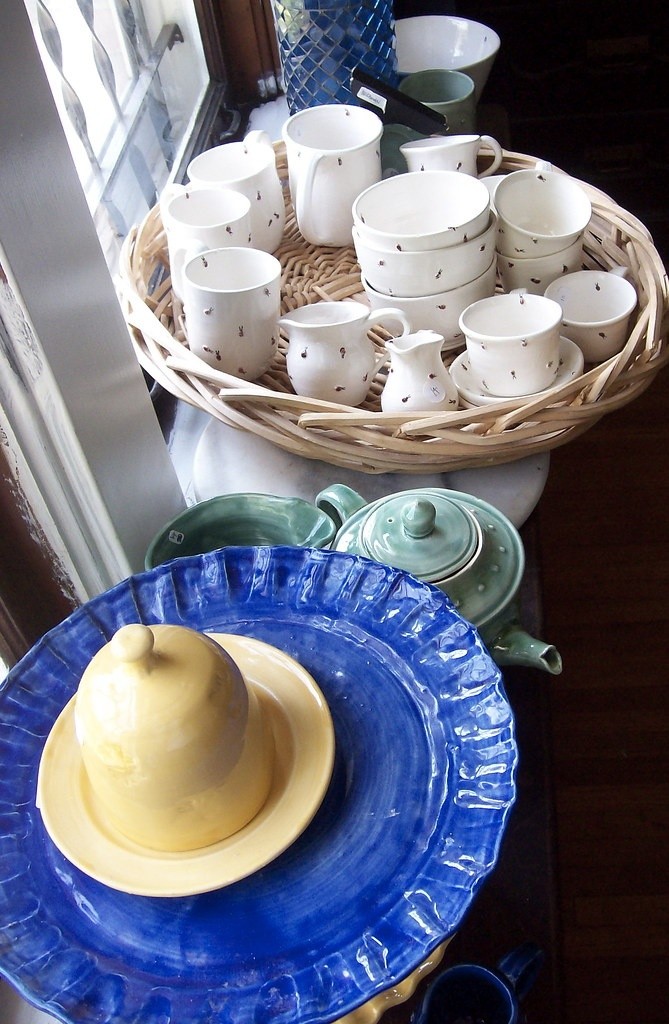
[350,168,497,352]
[386,14,502,104]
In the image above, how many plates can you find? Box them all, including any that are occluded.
[0,544,519,1024]
[450,335,583,411]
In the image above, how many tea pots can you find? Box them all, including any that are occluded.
[315,484,563,682]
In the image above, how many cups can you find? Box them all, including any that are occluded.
[400,134,502,178]
[271,0,404,135]
[142,491,336,572]
[412,945,542,1024]
[181,247,281,382]
[187,128,286,257]
[284,104,384,247]
[544,264,637,364]
[156,183,251,302]
[458,287,563,397]
[186,128,286,256]
[399,69,475,134]
[494,158,590,294]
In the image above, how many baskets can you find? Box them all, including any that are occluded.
[116,144,669,476]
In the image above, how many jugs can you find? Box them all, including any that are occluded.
[381,330,457,411]
[278,300,411,408]
